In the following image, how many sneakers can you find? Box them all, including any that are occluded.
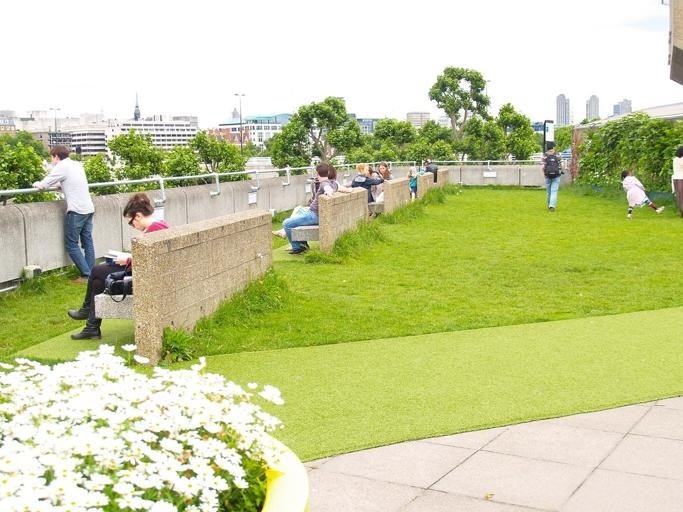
[272,228,287,240]
[655,205,664,213]
[627,214,631,218]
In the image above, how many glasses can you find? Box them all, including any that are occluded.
[128,216,135,228]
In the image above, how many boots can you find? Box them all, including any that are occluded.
[67,277,92,321]
[70,289,103,340]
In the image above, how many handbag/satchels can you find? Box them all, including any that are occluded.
[104,271,131,303]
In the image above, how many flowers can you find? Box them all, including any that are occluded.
[0,340,286,512]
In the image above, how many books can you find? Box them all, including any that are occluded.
[103,248,132,261]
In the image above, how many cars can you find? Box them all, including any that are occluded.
[560,148,571,159]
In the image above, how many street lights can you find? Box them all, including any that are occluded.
[48,106,63,149]
[541,119,554,152]
[233,93,247,157]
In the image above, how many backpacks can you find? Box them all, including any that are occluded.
[543,153,561,178]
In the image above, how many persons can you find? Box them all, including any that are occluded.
[620,170,665,220]
[541,140,566,212]
[64,191,172,341]
[30,146,98,285]
[272,159,439,255]
[672,146,683,217]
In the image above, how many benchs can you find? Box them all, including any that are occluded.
[94,207,272,365]
[291,167,450,256]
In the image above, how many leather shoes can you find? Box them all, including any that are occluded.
[70,276,89,283]
[289,249,302,254]
